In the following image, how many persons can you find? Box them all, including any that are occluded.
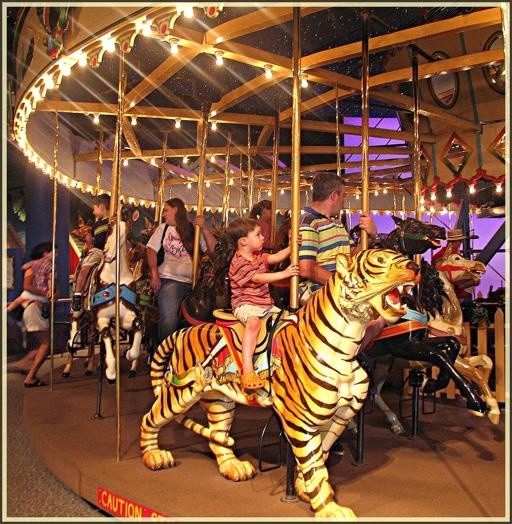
[348,224,466,350]
[14,241,63,387]
[71,193,303,390]
[299,170,378,307]
[7,242,60,314]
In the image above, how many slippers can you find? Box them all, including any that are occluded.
[6,367,47,388]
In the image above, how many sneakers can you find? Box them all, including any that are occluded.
[72,295,82,311]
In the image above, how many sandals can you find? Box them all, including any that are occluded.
[240,370,265,390]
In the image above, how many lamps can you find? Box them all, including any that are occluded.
[123,155,216,166]
[93,113,218,132]
[167,39,308,89]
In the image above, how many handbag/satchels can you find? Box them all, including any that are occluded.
[157,245,165,267]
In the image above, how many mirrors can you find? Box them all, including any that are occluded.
[427,50,459,109]
[481,30,505,95]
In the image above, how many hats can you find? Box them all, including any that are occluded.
[447,230,468,241]
